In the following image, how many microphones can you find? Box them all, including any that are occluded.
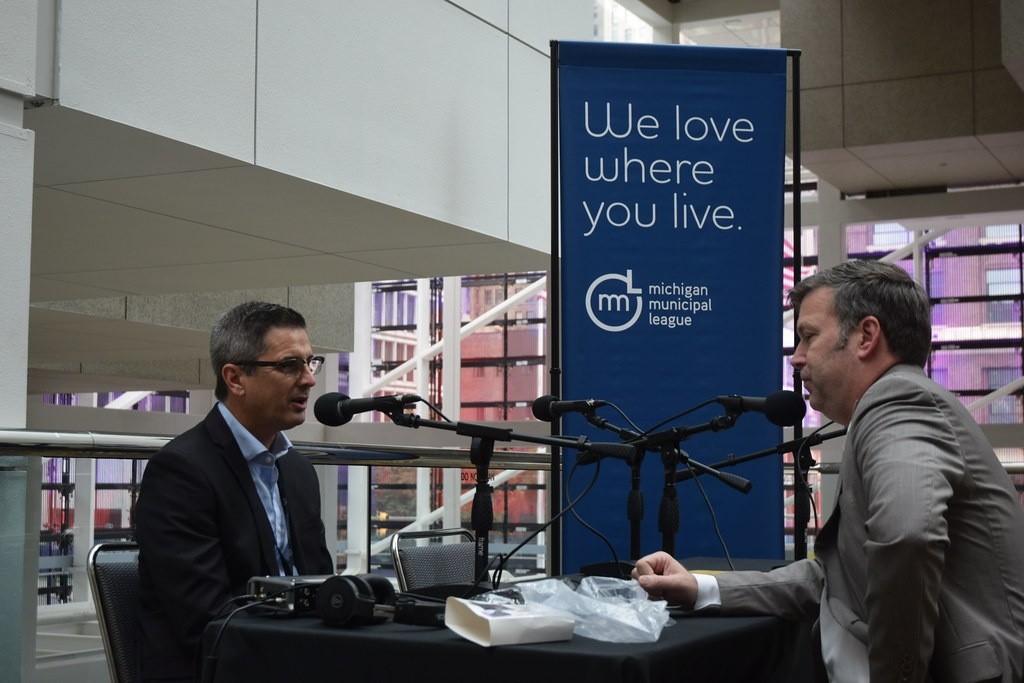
[532,394,607,422]
[313,392,421,427]
[715,391,807,427]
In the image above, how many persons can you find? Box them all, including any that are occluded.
[134,302,333,683]
[632,259,1024,683]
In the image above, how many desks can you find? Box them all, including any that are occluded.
[193,557,823,683]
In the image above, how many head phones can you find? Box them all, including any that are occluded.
[315,573,450,628]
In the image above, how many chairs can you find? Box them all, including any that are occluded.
[391,530,476,595]
[88,542,154,683]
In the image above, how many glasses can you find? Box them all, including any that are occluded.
[233,355,324,377]
